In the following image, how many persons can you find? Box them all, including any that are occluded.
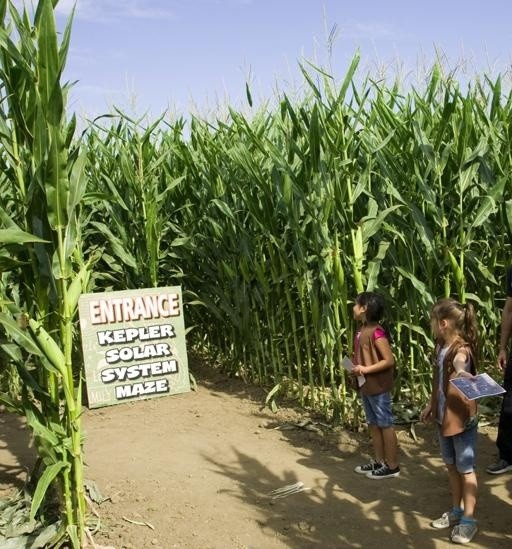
[483,263,511,476]
[347,290,402,482]
[418,294,479,545]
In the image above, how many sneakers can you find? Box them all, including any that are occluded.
[484,457,512,476]
[352,458,402,480]
[430,507,480,545]
